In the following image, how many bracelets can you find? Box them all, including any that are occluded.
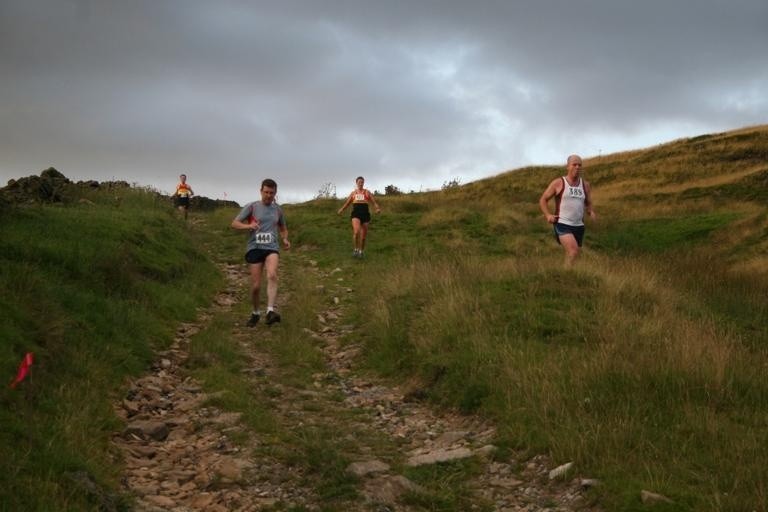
[376,208,380,209]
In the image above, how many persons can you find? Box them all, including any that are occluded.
[229,179,291,328]
[170,174,194,222]
[539,154,597,269]
[336,176,381,261]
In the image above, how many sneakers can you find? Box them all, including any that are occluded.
[353,248,363,259]
[246,313,260,329]
[265,311,280,325]
